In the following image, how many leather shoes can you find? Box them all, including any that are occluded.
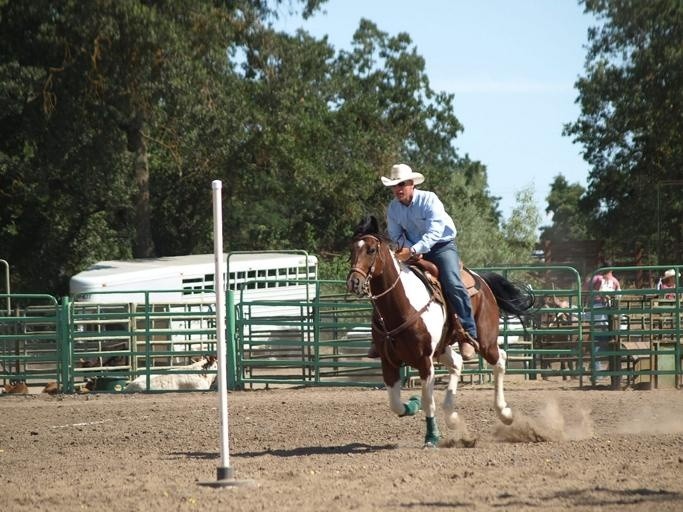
[368,343,381,358]
[459,335,475,360]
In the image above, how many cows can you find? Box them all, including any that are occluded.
[0,356,126,396]
[123,354,218,392]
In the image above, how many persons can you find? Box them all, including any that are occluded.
[584,266,621,327]
[366,163,478,361]
[660,268,681,300]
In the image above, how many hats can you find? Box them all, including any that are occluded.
[381,164,425,186]
[663,269,681,279]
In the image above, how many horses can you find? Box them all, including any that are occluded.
[536,291,591,380]
[345,215,534,449]
